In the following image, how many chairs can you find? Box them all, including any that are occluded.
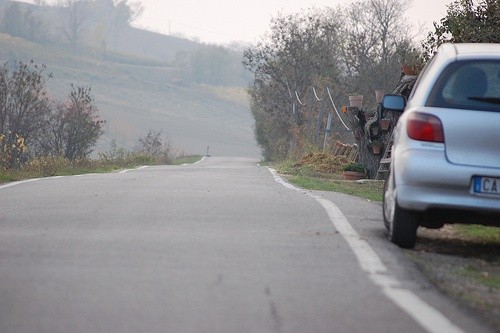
[451,67,488,101]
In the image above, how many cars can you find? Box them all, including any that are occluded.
[380,41,500,249]
